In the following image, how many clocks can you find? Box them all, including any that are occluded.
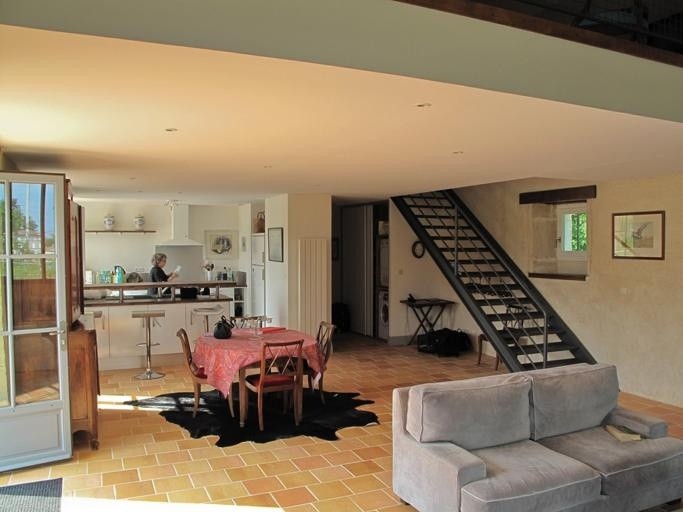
[412,240,427,258]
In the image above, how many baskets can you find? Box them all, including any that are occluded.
[254,217,265,233]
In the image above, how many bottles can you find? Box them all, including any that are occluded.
[94,266,125,284]
[222,266,232,281]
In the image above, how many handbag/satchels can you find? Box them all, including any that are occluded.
[214,320,231,339]
[452,332,472,353]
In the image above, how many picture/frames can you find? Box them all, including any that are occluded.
[268,227,284,264]
[611,209,665,261]
[204,230,240,265]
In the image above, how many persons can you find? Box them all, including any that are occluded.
[149,254,171,295]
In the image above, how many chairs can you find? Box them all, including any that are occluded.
[308,320,337,400]
[240,338,304,430]
[475,306,526,371]
[176,327,235,419]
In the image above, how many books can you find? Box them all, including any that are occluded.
[249,326,286,334]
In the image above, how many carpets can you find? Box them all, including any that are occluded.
[122,378,382,449]
[0,476,64,512]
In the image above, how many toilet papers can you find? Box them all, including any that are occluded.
[83,271,92,285]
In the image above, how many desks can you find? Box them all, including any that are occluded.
[400,294,456,346]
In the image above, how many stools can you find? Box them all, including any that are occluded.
[131,309,167,380]
[191,308,223,335]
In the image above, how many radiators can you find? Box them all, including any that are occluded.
[298,239,328,347]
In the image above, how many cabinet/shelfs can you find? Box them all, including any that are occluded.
[186,303,231,353]
[5,170,99,461]
[110,304,185,372]
[80,304,110,370]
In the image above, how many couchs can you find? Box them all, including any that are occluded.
[391,363,681,511]
